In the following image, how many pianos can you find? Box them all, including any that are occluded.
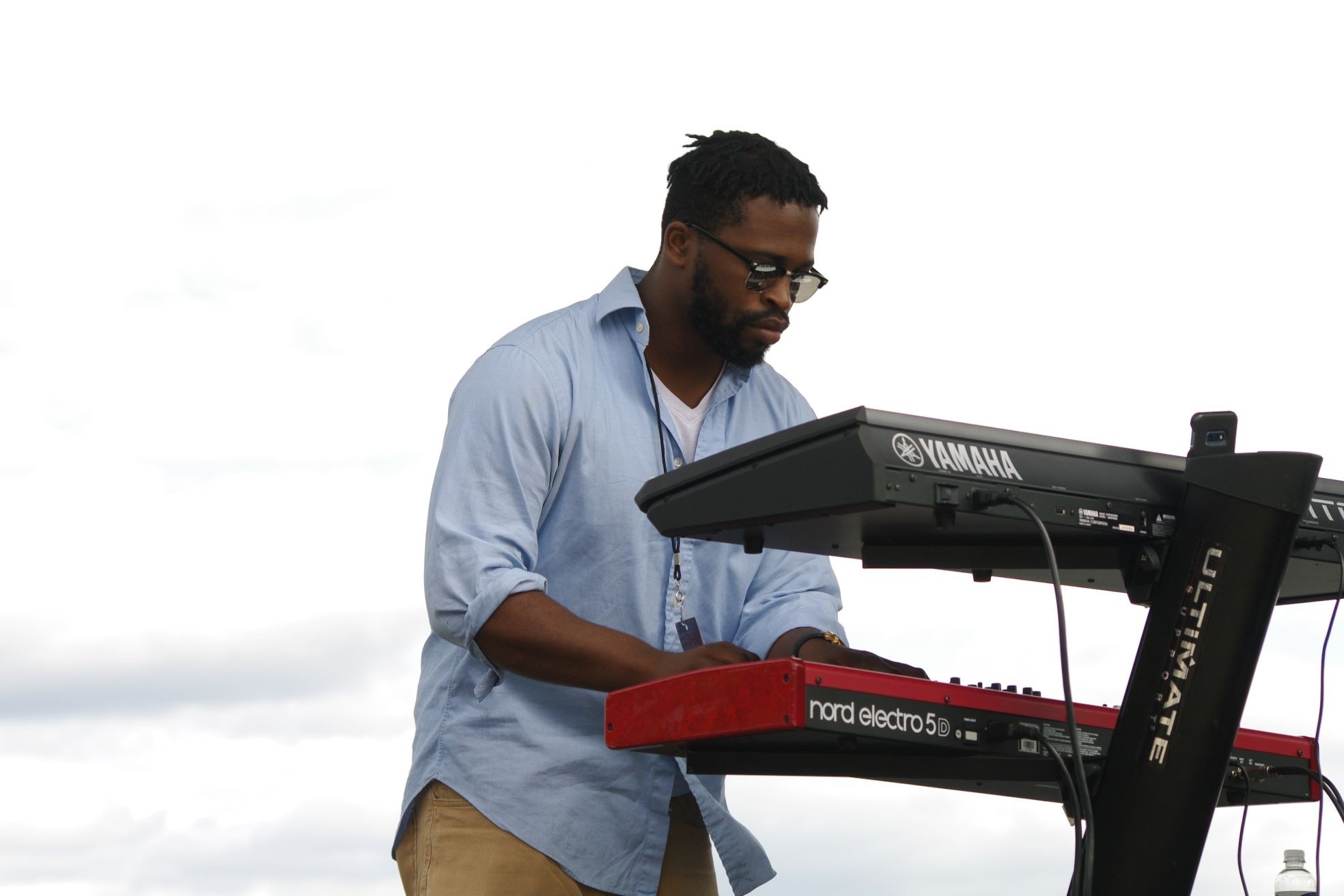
[604,395,1344,896]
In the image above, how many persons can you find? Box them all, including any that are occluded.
[391,130,850,896]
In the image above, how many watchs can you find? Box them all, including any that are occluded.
[792,631,847,658]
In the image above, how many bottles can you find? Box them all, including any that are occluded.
[1274,849,1317,896]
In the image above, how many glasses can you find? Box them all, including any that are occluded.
[691,221,828,306]
